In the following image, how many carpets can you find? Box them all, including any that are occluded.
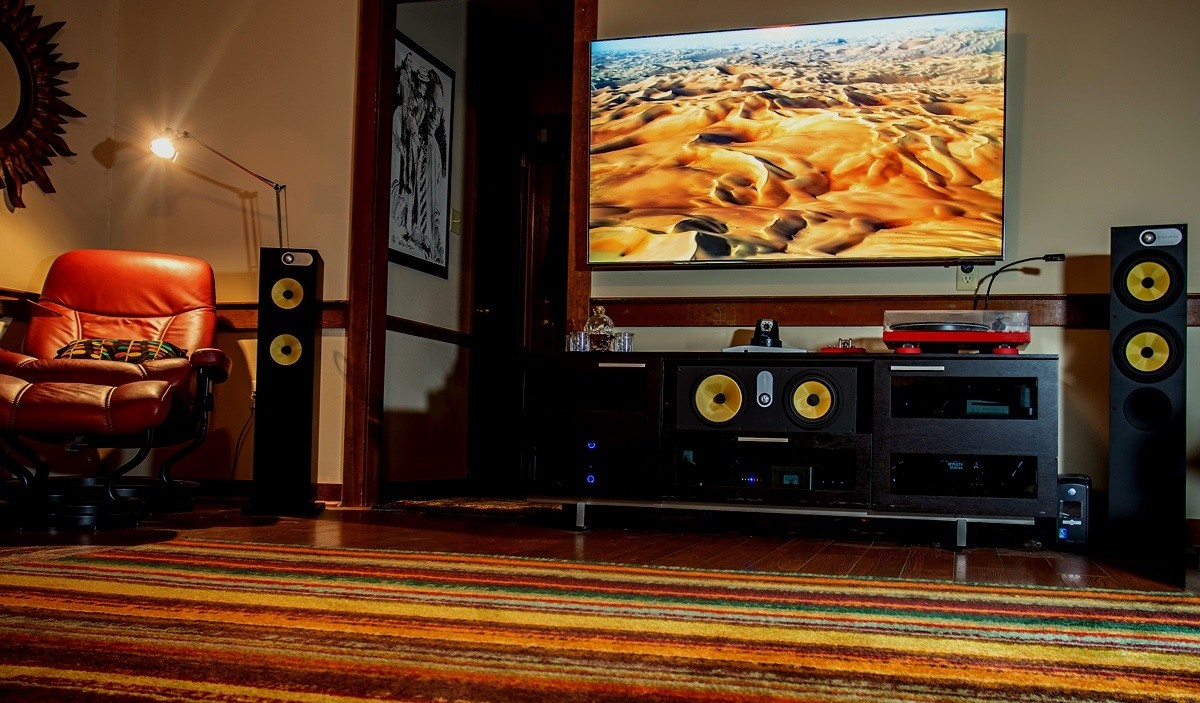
[0,531,1200,703]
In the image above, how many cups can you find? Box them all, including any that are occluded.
[615,332,632,351]
[570,332,590,351]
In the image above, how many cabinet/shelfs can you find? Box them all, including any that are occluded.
[495,346,1061,555]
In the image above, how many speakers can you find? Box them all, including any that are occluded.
[253,247,324,516]
[1104,223,1190,589]
[675,363,860,434]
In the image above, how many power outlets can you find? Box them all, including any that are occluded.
[955,265,980,291]
[249,379,257,403]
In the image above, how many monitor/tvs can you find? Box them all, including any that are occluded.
[585,8,1007,269]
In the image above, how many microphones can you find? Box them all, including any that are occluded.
[985,254,1065,309]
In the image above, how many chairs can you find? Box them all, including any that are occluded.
[0,247,234,539]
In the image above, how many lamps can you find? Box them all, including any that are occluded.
[146,121,293,251]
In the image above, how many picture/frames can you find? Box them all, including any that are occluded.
[387,28,459,282]
